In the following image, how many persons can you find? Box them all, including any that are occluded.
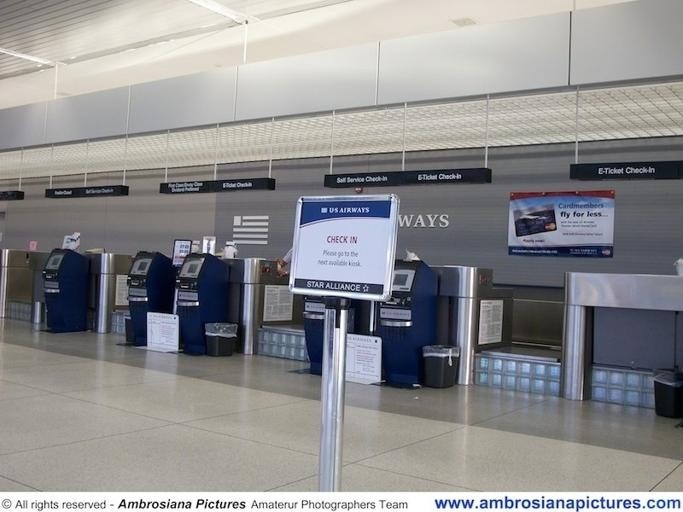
[278,248,292,277]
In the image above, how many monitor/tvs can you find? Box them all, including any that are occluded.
[178,257,207,278]
[44,252,66,270]
[128,257,154,277]
[392,267,417,292]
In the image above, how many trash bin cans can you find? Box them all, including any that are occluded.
[654,373,683,418]
[205,323,239,357]
[124,315,136,344]
[422,345,459,387]
[31,300,45,324]
